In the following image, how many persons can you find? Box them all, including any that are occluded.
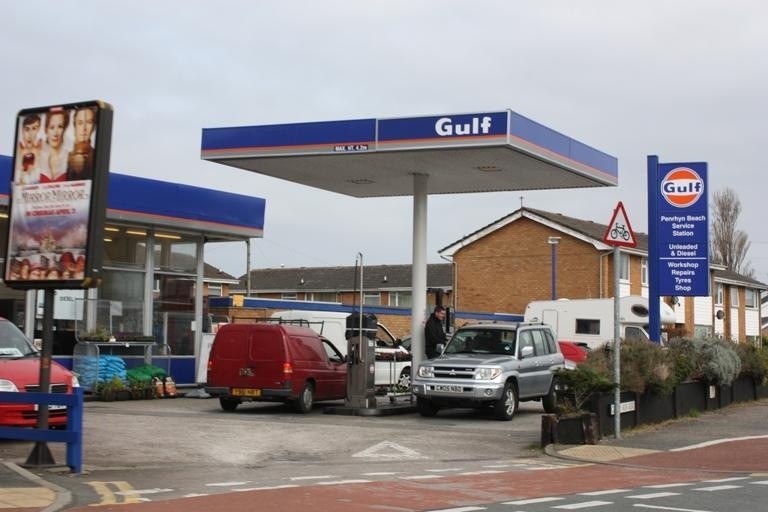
[424,306,450,359]
[8,106,95,280]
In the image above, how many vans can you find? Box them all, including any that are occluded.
[205,313,351,413]
[266,308,412,392]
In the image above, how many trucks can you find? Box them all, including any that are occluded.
[525,294,676,354]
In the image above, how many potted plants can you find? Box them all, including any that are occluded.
[98,374,129,400]
[130,379,157,400]
[78,326,110,343]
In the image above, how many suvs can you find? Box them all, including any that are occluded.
[415,320,564,420]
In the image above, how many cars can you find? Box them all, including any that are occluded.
[560,341,594,363]
[1,316,81,435]
[398,332,468,353]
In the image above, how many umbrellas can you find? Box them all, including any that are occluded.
[559,340,591,362]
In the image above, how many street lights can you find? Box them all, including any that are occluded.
[546,236,561,300]
